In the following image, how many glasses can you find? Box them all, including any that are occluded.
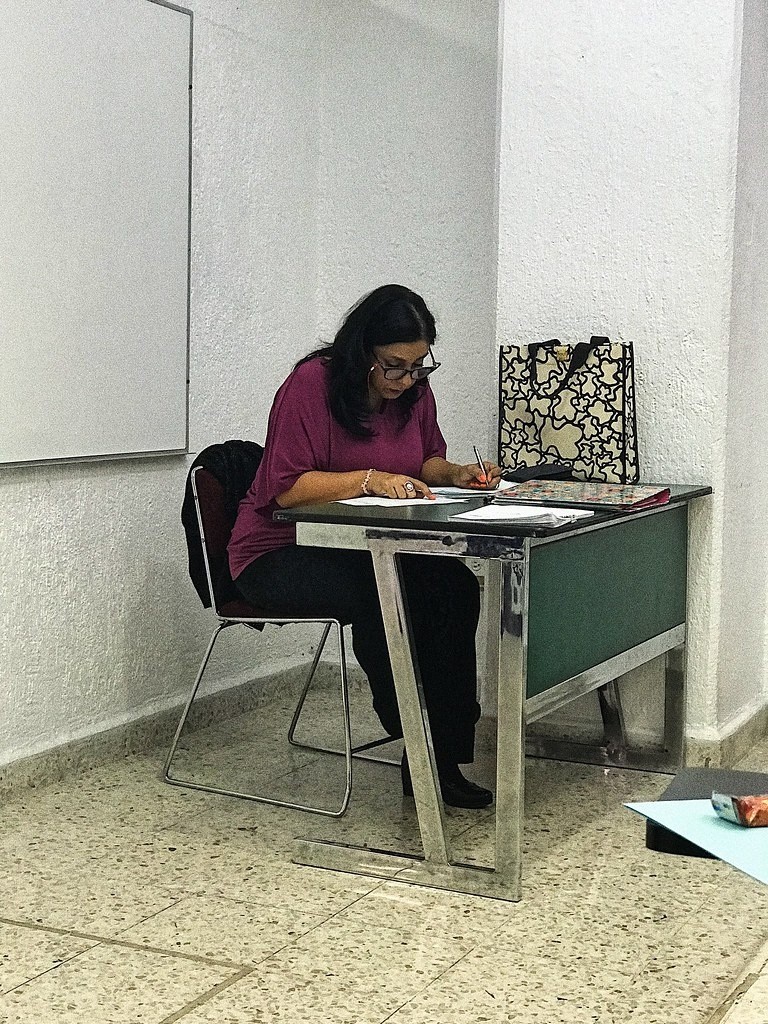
[372,346,442,379]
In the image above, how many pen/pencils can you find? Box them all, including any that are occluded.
[473,445,489,487]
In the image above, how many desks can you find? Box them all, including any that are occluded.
[272,479,714,904]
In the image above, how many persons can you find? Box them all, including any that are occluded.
[221,282,497,809]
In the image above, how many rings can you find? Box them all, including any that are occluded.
[404,481,414,491]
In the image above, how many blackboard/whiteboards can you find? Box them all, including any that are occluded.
[0,0,194,470]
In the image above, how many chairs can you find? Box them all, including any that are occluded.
[161,440,401,818]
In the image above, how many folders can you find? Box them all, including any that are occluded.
[487,479,672,513]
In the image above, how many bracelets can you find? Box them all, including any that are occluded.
[361,468,376,496]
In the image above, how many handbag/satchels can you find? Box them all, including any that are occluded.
[498,336,640,486]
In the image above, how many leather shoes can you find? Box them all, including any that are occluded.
[401,754,493,809]
